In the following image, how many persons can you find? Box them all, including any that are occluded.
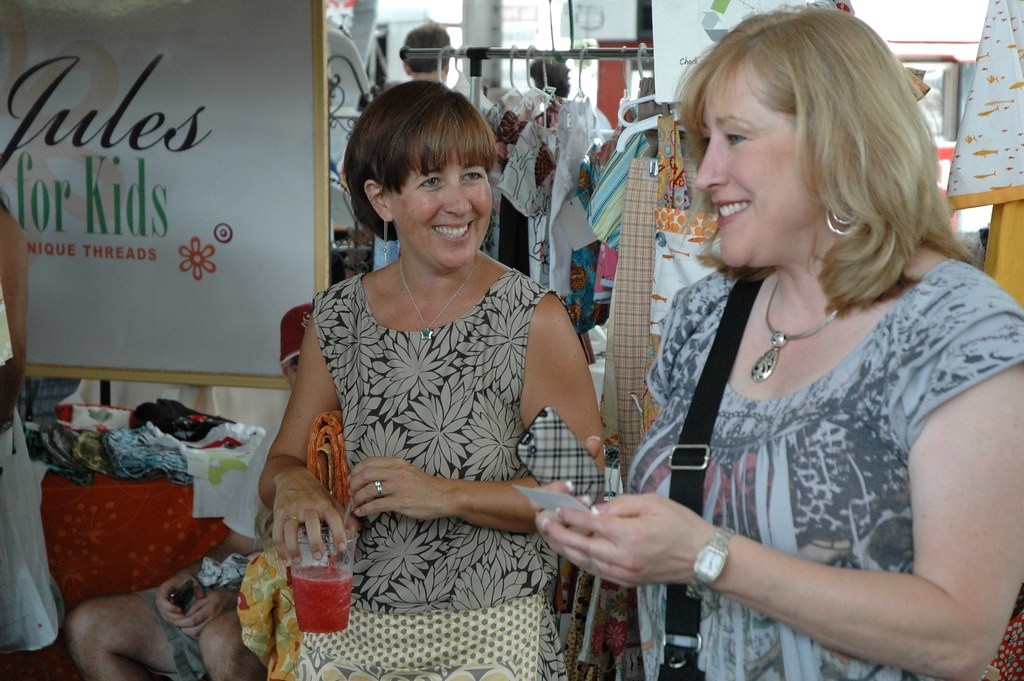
[0,191,66,652]
[530,57,570,99]
[405,24,451,84]
[259,80,605,681]
[540,5,1023,681]
[65,303,317,681]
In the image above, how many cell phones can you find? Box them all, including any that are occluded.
[170,579,194,609]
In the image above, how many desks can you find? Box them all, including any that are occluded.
[38,455,230,613]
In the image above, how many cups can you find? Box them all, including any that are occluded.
[291,527,359,633]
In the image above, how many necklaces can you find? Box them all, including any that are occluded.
[399,256,477,340]
[751,269,837,383]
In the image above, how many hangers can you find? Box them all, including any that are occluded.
[434,43,684,156]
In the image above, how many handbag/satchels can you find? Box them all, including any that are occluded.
[0,406,58,655]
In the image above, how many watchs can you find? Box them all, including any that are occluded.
[685,527,735,598]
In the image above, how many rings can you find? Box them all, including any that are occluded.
[283,514,296,522]
[374,481,382,497]
